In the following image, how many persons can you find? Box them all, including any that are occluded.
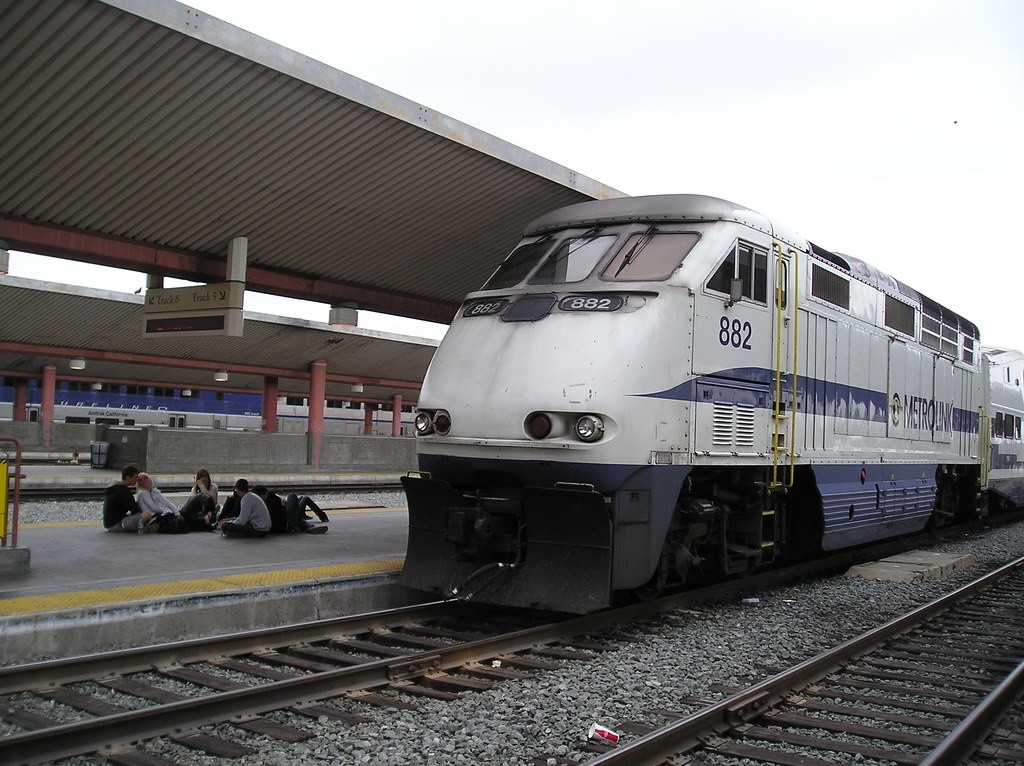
[101,466,330,536]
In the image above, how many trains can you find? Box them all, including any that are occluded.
[0,375,417,437]
[400,198,1024,616]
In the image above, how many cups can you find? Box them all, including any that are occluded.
[588,722,619,745]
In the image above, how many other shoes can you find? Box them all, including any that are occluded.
[309,526,328,534]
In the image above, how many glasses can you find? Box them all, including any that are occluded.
[200,474,208,478]
